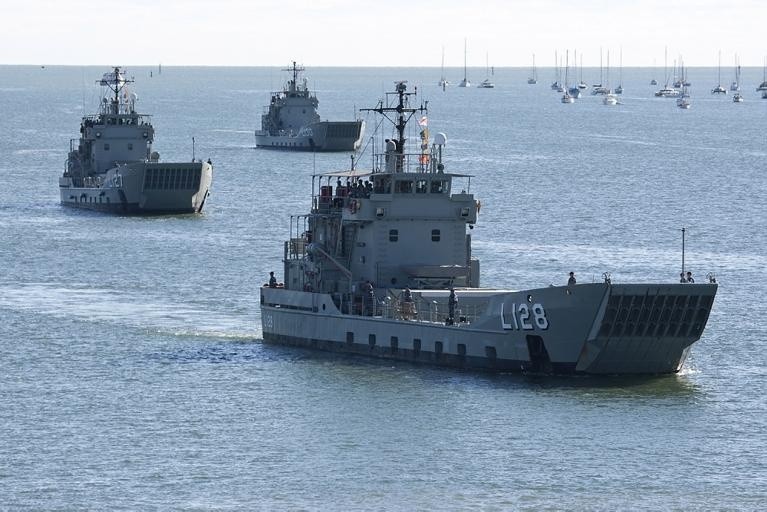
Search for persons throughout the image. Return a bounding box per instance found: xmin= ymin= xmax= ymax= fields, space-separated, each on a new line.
xmin=678 ymin=273 xmax=685 ymax=283
xmin=404 ymin=284 xmax=412 ymax=301
xmin=384 ymin=138 xmax=397 ymax=162
xmin=334 ymin=179 xmax=384 ymax=211
xmin=268 ymin=272 xmax=277 ymax=307
xmin=567 ymin=271 xmax=576 ymax=285
xmin=365 ymin=281 xmax=373 ymax=296
xmin=686 ymin=272 xmax=694 ymax=284
xmin=446 ymin=288 xmax=458 ymax=319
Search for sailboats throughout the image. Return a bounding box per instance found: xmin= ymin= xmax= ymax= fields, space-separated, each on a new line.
xmin=527 ymin=48 xmax=624 ymax=107
xmin=438 ymin=39 xmax=495 ymax=89
xmin=715 ymin=49 xmax=766 ymax=104
xmin=650 ymin=47 xmax=692 ymax=110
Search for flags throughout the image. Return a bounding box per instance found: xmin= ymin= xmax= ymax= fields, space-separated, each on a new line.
xmin=419 ymin=115 xmax=429 ymax=166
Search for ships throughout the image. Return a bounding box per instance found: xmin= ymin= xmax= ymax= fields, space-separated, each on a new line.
xmin=253 ymin=75 xmax=726 ymax=384
xmin=252 ymin=60 xmax=366 ymax=152
xmin=55 ymin=63 xmax=217 ymax=218
